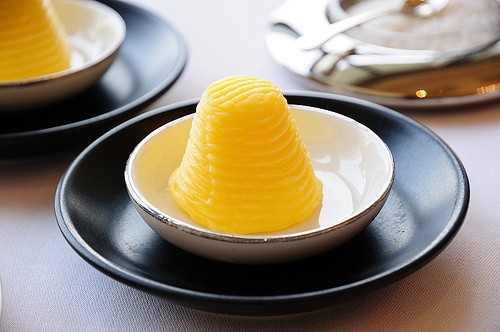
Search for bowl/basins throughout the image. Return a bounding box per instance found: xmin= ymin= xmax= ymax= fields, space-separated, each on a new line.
xmin=0 ymin=0 xmax=127 ymax=109
xmin=320 ymin=0 xmax=500 ymax=74
xmin=52 ymin=88 xmax=470 ymax=318
xmin=123 ymin=103 xmax=396 ymax=268
xmin=0 ymin=0 xmax=188 ymax=140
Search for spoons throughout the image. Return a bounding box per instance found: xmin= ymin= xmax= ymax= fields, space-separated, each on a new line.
xmin=292 ymin=0 xmax=453 ymax=53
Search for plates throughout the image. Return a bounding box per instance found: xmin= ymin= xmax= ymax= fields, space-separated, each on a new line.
xmin=256 ymin=0 xmax=500 ymax=110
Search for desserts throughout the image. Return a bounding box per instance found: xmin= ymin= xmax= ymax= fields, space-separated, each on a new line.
xmin=123 ymin=75 xmax=397 ymax=261
xmin=0 ymin=0 xmax=126 ymax=106
